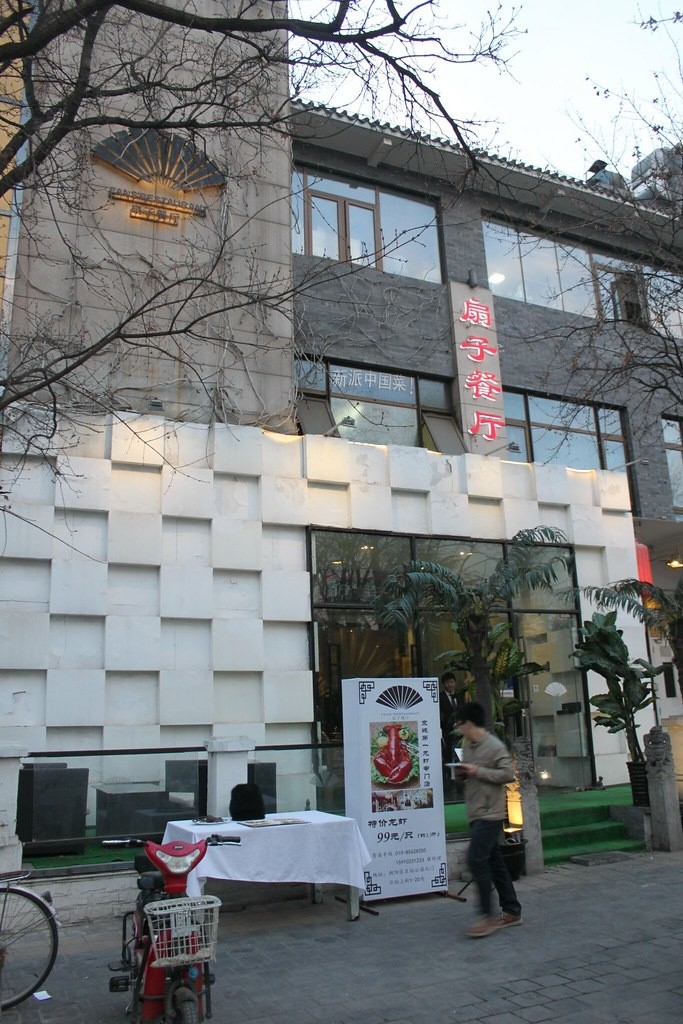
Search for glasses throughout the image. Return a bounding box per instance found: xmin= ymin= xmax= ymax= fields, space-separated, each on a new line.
xmin=453 ymin=722 xmax=464 ymax=729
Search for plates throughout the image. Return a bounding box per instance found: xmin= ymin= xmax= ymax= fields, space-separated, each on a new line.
xmin=190 ymin=818 xmax=229 ymax=825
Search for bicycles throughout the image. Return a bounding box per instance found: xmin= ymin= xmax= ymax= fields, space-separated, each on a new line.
xmin=0 ymin=867 xmax=64 ymax=1011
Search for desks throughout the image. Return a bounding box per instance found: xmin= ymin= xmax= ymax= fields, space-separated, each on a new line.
xmin=160 ymin=809 xmax=371 ymax=922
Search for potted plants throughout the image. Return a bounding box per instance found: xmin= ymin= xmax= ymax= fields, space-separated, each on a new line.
xmin=566 ymin=607 xmax=677 ymax=809
xmin=377 ymin=525 xmax=575 ymax=884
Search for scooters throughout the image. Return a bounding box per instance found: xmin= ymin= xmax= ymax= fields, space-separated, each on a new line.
xmin=102 ymin=830 xmax=242 ymax=1024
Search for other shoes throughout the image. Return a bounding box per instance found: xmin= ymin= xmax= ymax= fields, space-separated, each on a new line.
xmin=496 ymin=911 xmax=523 ymax=928
xmin=458 ymin=793 xmax=464 ymax=800
xmin=465 ymin=915 xmax=497 ymax=936
xmin=445 ymin=792 xmax=452 ymax=801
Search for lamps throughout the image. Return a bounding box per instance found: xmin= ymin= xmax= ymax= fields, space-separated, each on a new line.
xmin=466 ymin=269 xmax=478 ymax=287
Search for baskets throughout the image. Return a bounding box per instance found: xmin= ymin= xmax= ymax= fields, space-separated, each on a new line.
xmin=143 ymin=895 xmax=222 ymax=966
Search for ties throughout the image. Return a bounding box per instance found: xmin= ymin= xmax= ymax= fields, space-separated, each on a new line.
xmin=450 ymin=694 xmax=455 ymax=706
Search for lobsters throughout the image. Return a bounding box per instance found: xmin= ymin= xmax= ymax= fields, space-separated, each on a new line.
xmin=374 ymin=724 xmax=413 ymax=783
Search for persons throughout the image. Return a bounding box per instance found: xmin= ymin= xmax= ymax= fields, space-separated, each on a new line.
xmin=454 ymin=701 xmax=524 ymax=937
xmin=439 ymin=672 xmax=464 ymax=801
